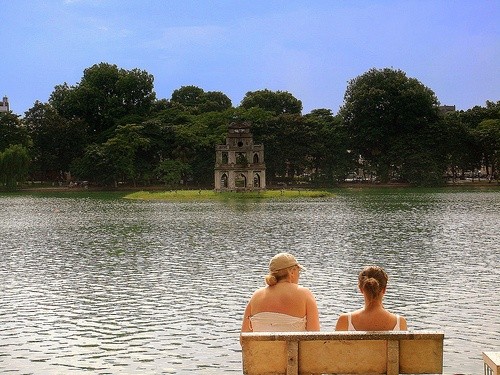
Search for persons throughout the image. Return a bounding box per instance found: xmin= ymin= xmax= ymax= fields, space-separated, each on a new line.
xmin=240 ymin=253 xmax=320 ymax=346
xmin=335 ymin=265 xmax=407 ymax=331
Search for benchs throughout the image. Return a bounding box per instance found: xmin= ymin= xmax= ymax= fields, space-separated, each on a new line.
xmin=240 ymin=330 xmax=444 ymax=375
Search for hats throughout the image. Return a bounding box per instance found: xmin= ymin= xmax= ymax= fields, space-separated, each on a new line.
xmin=269 ymin=253 xmax=307 ymax=273
